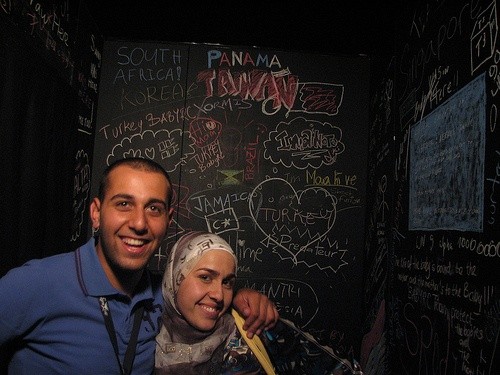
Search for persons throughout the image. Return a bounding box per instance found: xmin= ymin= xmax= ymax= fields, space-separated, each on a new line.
xmin=0 ymin=156 xmax=279 ymax=375
xmin=154 ymin=230 xmax=363 ymax=375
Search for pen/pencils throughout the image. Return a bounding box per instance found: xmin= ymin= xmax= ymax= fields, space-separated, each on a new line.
xmin=143 ymin=316 xmax=155 ymax=331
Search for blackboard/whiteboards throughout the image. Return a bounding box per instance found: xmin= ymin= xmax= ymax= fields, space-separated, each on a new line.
xmin=1 ymin=0 xmax=500 ymax=375
